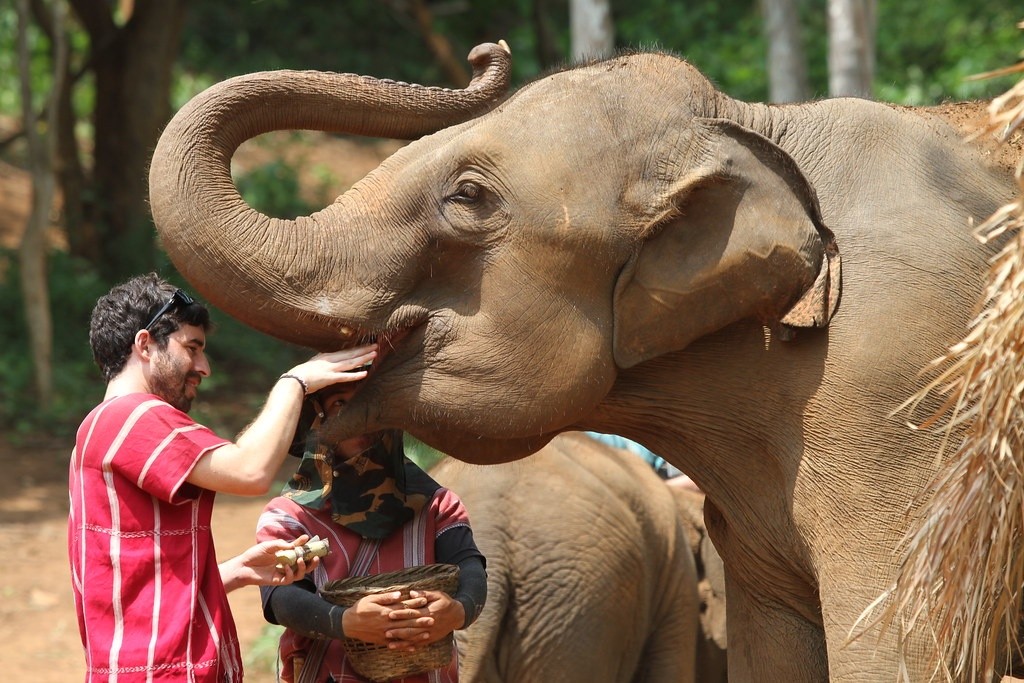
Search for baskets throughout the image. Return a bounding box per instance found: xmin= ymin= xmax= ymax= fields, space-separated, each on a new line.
xmin=318 ymin=563 xmax=460 ymax=683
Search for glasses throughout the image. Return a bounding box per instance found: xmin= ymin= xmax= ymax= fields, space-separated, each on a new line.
xmin=134 ymin=288 xmax=194 ymax=344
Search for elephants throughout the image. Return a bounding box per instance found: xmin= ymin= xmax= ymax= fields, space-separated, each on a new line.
xmin=149 ymin=37 xmax=1021 ymax=682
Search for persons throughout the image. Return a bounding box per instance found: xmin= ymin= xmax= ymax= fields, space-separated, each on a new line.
xmin=70 ymin=272 xmax=377 ymax=683
xmin=257 ymin=365 xmax=488 ymax=683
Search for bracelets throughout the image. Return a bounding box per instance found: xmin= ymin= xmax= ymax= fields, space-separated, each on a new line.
xmin=280 ymin=373 xmax=308 ymax=398
